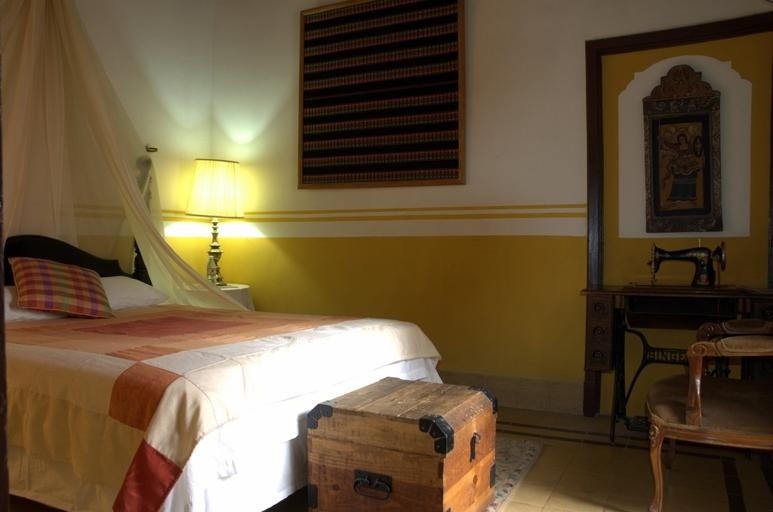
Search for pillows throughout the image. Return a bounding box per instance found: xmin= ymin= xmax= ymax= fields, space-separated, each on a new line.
xmin=8 ymin=256 xmax=118 ymax=319
xmin=4 ymin=285 xmax=69 ymax=322
xmin=99 ymin=275 xmax=171 ymax=312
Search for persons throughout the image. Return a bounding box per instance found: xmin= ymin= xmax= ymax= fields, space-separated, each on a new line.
xmin=661 ymin=123 xmax=705 ymax=203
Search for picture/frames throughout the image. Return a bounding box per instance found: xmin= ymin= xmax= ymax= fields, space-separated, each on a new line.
xmin=642 ymin=64 xmax=723 ymax=233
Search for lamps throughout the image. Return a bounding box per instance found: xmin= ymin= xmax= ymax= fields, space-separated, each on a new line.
xmin=183 ymin=158 xmax=246 ymax=286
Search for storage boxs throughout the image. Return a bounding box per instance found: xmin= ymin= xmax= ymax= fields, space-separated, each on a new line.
xmin=306 ymin=376 xmax=498 ymax=512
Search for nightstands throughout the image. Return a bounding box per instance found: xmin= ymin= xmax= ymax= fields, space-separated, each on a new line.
xmin=202 ymin=284 xmax=256 ymax=313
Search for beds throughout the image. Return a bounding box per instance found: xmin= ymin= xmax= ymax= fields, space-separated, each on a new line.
xmin=0 ymin=234 xmax=443 ymax=512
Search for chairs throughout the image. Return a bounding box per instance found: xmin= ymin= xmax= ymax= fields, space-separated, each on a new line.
xmin=644 ymin=316 xmax=773 ymax=512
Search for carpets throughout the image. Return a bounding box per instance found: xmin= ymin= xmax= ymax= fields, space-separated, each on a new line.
xmin=482 ymin=428 xmax=544 ymax=512
